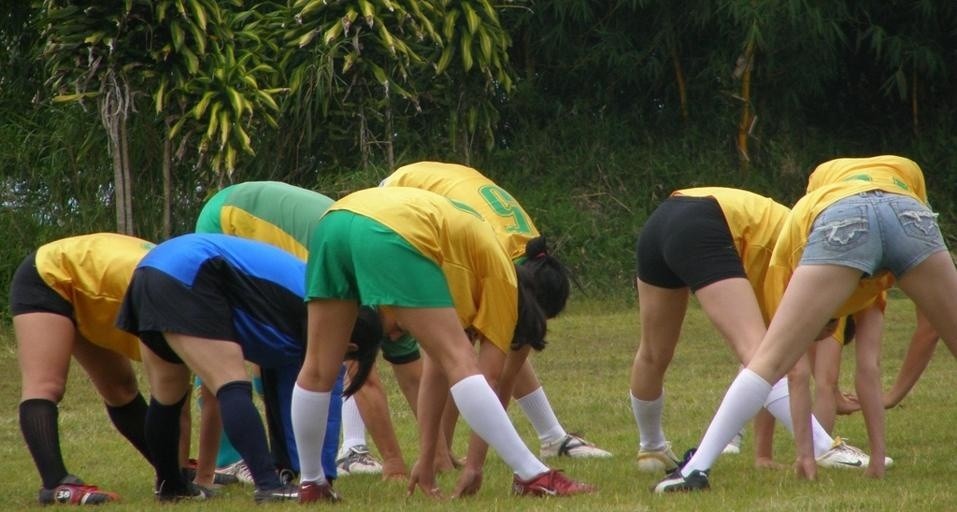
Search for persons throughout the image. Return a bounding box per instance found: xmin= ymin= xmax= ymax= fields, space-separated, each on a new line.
xmin=286 ymin=180 xmax=601 ymax=502
xmin=7 ymin=232 xmax=238 ymax=504
xmin=625 ymin=189 xmax=894 ymax=474
xmin=805 ymin=154 xmax=940 ymax=416
xmin=646 ymin=181 xmax=956 ymax=496
xmin=111 ymin=233 xmax=382 ymax=501
xmin=375 ymin=158 xmax=614 ymax=472
xmin=192 ymin=176 xmax=457 ymax=485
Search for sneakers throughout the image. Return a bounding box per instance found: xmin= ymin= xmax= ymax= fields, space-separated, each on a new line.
xmin=637 ymin=441 xmax=681 ymax=472
xmin=215 ymin=460 xmax=252 ymax=481
xmin=180 ymin=465 xmax=238 ymax=484
xmin=511 ymin=469 xmax=596 ymax=496
xmin=540 ymin=433 xmax=610 ymax=457
xmin=38 ymin=475 xmax=119 ymax=504
xmin=652 ymin=448 xmax=711 ymax=492
xmin=298 ymin=478 xmax=340 ymax=502
xmin=815 ymin=436 xmax=894 ymax=467
xmin=155 ymin=480 xmax=211 ymax=500
xmin=255 ymin=484 xmax=300 ymax=502
xmin=336 ymin=445 xmax=382 ymax=473
